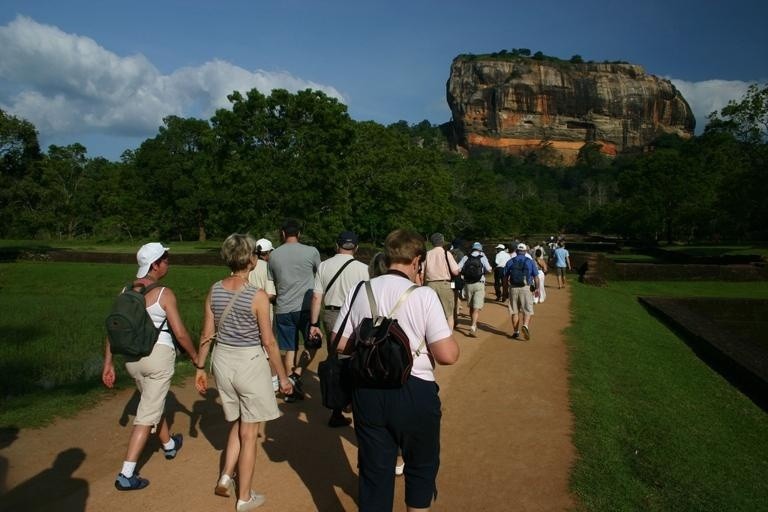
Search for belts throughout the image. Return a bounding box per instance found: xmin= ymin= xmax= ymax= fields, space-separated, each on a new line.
xmin=428 ymin=280 xmax=451 ymax=281
xmin=325 ymin=306 xmax=340 ymax=310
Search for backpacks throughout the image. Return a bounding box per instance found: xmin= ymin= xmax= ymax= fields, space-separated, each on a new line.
xmin=337 ymin=282 xmax=426 ymax=389
xmin=106 ymin=281 xmax=167 ymax=357
xmin=510 ymin=258 xmax=529 ymax=286
xmin=462 ymin=254 xmax=484 ymax=282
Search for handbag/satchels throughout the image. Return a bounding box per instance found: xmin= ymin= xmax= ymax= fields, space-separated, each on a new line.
xmin=318 ymin=355 xmax=351 ymax=409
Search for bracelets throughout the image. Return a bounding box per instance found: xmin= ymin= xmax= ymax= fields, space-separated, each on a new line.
xmin=309 ymin=322 xmax=321 ymax=327
xmin=196 ymin=364 xmax=206 ymax=370
xmin=279 ymin=379 xmax=290 ymax=385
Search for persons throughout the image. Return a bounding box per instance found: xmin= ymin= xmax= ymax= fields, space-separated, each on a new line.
xmin=228 ymin=237 xmax=278 ymax=362
xmin=327 ymin=227 xmax=462 ymax=512
xmin=394 ymin=448 xmax=408 ymax=476
xmin=266 ymin=221 xmax=322 ymax=402
xmin=193 ymin=231 xmax=296 ymax=511
xmin=101 ymin=241 xmax=201 ymax=491
xmin=367 ymin=231 xmax=572 ymax=339
xmin=308 ymin=229 xmax=370 ymax=427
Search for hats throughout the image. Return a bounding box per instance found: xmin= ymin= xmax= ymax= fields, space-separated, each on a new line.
xmin=472 ymin=242 xmax=482 ymax=250
xmin=337 ymin=232 xmax=357 ymax=250
xmin=517 ymin=243 xmax=527 ymax=250
xmin=136 ymin=242 xmax=169 ymax=278
xmin=256 ymin=238 xmax=274 ymax=251
xmin=494 ymin=244 xmax=505 ymax=249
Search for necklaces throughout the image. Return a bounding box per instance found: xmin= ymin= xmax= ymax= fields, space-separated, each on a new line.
xmin=230 ymin=272 xmax=250 ymax=282
xmin=142 ymin=274 xmax=157 ymax=282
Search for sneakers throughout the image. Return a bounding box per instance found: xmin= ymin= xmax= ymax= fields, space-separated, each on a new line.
xmin=164 ymin=434 xmax=182 ymax=459
xmin=329 ymin=414 xmax=352 ymax=427
xmin=215 ymin=474 xmax=232 ymax=497
xmin=272 ymin=374 xmax=304 ymax=402
xmin=470 ymin=324 xmax=478 ymax=336
xmin=115 ymin=473 xmax=149 ymax=490
xmin=237 ymin=494 xmax=264 ymax=511
xmin=513 ymin=326 xmax=529 ymax=340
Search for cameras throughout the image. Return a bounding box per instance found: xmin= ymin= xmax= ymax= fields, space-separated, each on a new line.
xmin=300 ymin=333 xmax=322 ymax=367
